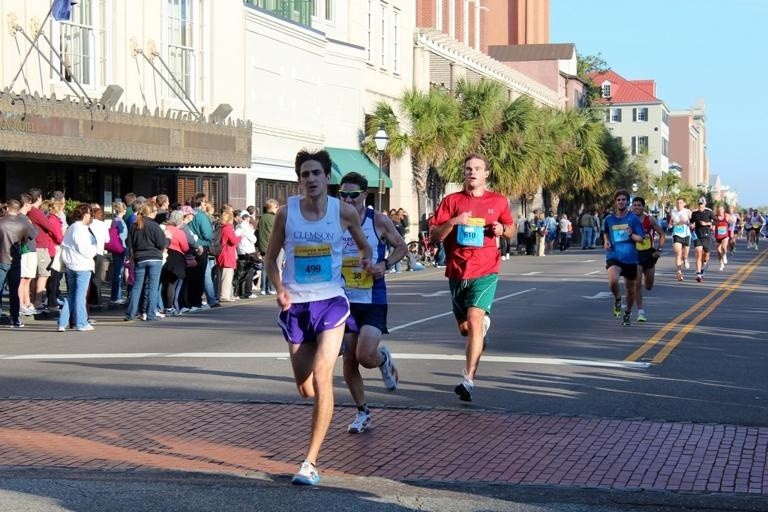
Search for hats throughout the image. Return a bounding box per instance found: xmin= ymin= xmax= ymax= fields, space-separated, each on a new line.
xmin=697 ymin=196 xmax=707 ymax=205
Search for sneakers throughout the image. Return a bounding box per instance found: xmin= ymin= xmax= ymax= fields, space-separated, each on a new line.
xmin=453 ymin=376 xmax=475 ymax=403
xmin=613 ymin=302 xmax=622 ymax=319
xmin=376 ymin=347 xmax=401 ymax=394
xmin=622 ymin=310 xmax=632 ymax=326
xmin=0 ymin=282 xmax=277 ymax=332
xmin=500 ymin=240 xmax=599 ymax=262
xmin=480 ymin=315 xmax=491 ymax=338
xmin=673 ymin=234 xmax=759 ymax=282
xmin=388 ymin=259 xmax=447 ymax=274
xmin=637 ymin=310 xmax=648 ymax=322
xmin=347 ymin=409 xmax=372 ymax=435
xmin=288 ymin=459 xmax=320 ymax=486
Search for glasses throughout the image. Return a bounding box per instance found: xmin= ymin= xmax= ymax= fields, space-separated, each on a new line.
xmin=337 ymin=188 xmax=365 ymax=199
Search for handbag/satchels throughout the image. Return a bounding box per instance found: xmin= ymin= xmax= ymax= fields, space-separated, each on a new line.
xmin=182 ymin=253 xmax=198 ymax=267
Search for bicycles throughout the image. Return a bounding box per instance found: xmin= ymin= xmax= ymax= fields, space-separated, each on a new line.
xmin=406 ymin=230 xmax=437 ymax=261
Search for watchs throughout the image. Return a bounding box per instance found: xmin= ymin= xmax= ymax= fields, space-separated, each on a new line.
xmin=382 ymin=258 xmax=391 ymax=271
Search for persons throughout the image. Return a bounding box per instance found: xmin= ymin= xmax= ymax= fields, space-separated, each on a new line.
xmin=262 ymin=144 xmax=374 ymax=485
xmin=1 ymin=187 xmax=283 ymax=333
xmin=334 ymin=172 xmax=408 ymax=434
xmin=428 ymin=152 xmax=517 ymax=403
xmin=381 ymin=204 xmax=601 ymax=277
xmin=601 ymin=187 xmax=768 ymax=326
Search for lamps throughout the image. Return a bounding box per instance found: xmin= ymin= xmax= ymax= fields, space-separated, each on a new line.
xmin=208 ymin=103 xmax=233 ymax=125
xmin=99 ymin=84 xmax=124 ymax=111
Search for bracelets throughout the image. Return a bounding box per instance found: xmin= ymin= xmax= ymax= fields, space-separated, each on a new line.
xmin=360 ymin=256 xmax=367 ymax=260
xmin=501 ymin=225 xmax=506 ymax=234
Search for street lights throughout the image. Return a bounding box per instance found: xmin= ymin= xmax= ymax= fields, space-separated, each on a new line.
xmin=653 ymin=186 xmax=659 ymax=213
xmin=632 ymin=182 xmax=638 ymax=198
xmin=372 ymin=127 xmax=392 ymax=213
